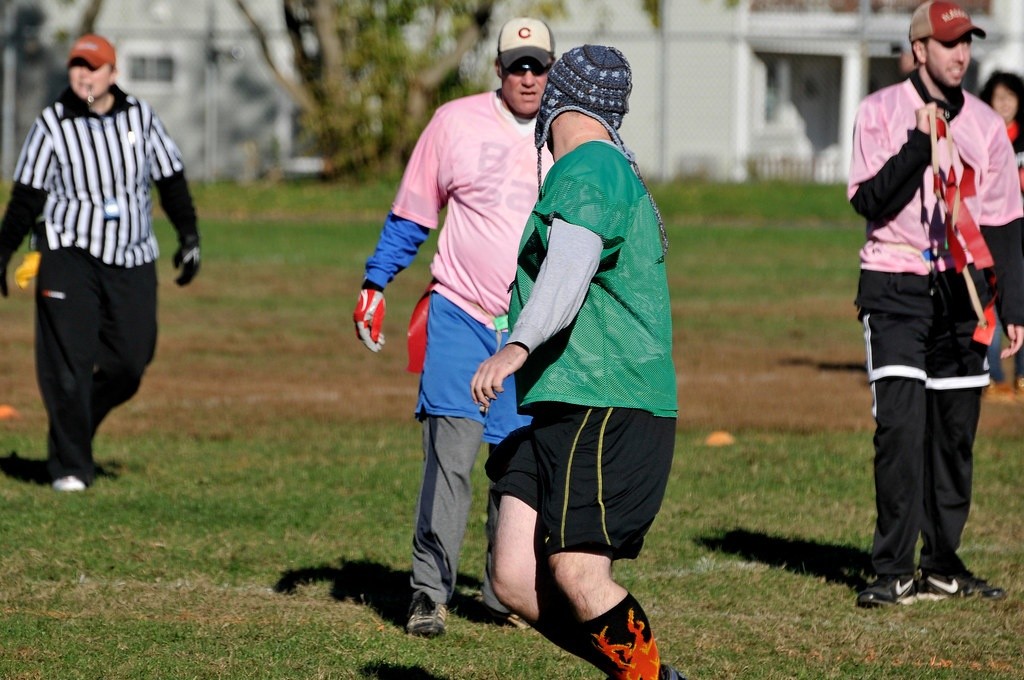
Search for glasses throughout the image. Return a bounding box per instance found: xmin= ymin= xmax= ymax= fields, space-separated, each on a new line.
xmin=506 ymin=57 xmax=551 ymax=76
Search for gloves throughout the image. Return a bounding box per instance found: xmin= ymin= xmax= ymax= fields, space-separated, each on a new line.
xmin=351 ymin=276 xmax=386 ymax=353
xmin=173 ymin=236 xmax=201 ymax=286
xmin=0 ymin=248 xmax=8 ymax=298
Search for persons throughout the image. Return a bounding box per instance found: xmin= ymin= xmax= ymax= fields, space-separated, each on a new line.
xmin=351 ymin=18 xmax=554 ymax=642
xmin=0 ymin=32 xmax=203 ymax=493
xmin=846 ymin=0 xmax=1024 ymax=609
xmin=470 ymin=44 xmax=691 ymax=680
xmin=969 ymin=70 xmax=1024 ymax=401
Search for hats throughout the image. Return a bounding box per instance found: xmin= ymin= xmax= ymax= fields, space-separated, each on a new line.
xmin=535 ymin=45 xmax=671 ymax=263
xmin=909 ymin=0 xmax=986 ymax=43
xmin=497 ymin=16 xmax=555 ymax=69
xmin=67 ymin=33 xmax=116 ymax=69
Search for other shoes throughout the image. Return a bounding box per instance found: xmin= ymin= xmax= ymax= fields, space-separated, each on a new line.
xmin=658 ymin=664 xmax=687 ymax=680
xmin=51 ymin=476 xmax=87 ymax=492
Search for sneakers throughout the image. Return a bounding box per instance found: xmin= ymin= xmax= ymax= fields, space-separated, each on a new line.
xmin=483 ymin=597 xmax=533 ymax=629
xmin=856 ymin=575 xmax=918 ymax=606
xmin=405 ymin=596 xmax=448 ymax=639
xmin=916 ymin=564 xmax=1006 ymax=605
